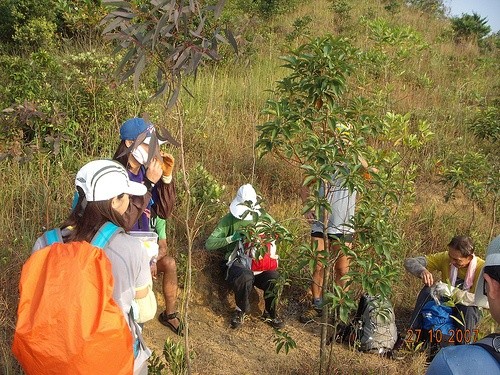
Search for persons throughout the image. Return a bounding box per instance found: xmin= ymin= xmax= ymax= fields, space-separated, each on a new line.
xmin=113 ymin=117 xmax=193 ymax=338
xmin=27 ymin=159 xmax=159 ymax=375
xmin=424 ymin=236 xmax=500 ymax=375
xmin=385 ymin=234 xmax=488 ymax=365
xmin=297 ymin=119 xmax=379 ymax=323
xmin=203 ymin=183 xmax=287 ymax=329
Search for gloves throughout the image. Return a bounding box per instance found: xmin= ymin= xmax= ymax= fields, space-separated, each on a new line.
xmin=226 ymin=231 xmax=242 ymax=244
xmin=159 ymin=151 xmax=175 ymax=175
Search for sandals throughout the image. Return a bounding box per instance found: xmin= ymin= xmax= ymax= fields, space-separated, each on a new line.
xmin=158 ymin=310 xmax=190 ymax=336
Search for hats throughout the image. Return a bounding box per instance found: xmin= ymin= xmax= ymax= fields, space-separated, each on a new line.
xmin=334 ymin=123 xmax=355 ymax=148
xmin=229 ymin=183 xmax=262 ymax=220
xmin=119 ymin=117 xmax=166 ymax=146
xmin=73 ymin=160 xmax=147 ymax=201
xmin=473 ymin=235 xmax=500 ymax=305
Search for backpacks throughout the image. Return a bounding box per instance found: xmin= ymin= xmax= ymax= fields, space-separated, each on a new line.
xmin=227 ymin=222 xmax=279 ymax=271
xmin=11 ymin=222 xmax=133 ymax=375
xmin=352 ymin=295 xmax=397 ymax=355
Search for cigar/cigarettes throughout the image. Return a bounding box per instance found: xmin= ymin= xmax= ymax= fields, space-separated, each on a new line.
xmin=153 ymin=276 xmax=158 ymax=281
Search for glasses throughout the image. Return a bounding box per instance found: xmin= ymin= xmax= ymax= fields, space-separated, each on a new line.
xmin=483 ymin=279 xmax=487 ymax=297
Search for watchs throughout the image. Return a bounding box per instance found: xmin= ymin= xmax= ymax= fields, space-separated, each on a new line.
xmin=151 ymin=258 xmax=159 ymax=264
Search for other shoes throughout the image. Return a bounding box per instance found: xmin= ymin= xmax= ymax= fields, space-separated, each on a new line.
xmin=393 ymin=344 xmax=417 ymax=359
xmin=425 ymin=348 xmax=438 ymax=367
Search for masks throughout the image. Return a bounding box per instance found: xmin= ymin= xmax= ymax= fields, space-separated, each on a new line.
xmin=127 ymin=140 xmax=149 ymax=165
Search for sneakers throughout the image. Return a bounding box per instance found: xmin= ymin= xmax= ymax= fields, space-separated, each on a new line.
xmin=261 ymin=309 xmax=284 ymax=329
xmin=299 ymin=304 xmax=322 ymax=324
xmin=229 ymin=305 xmax=247 ymax=328
